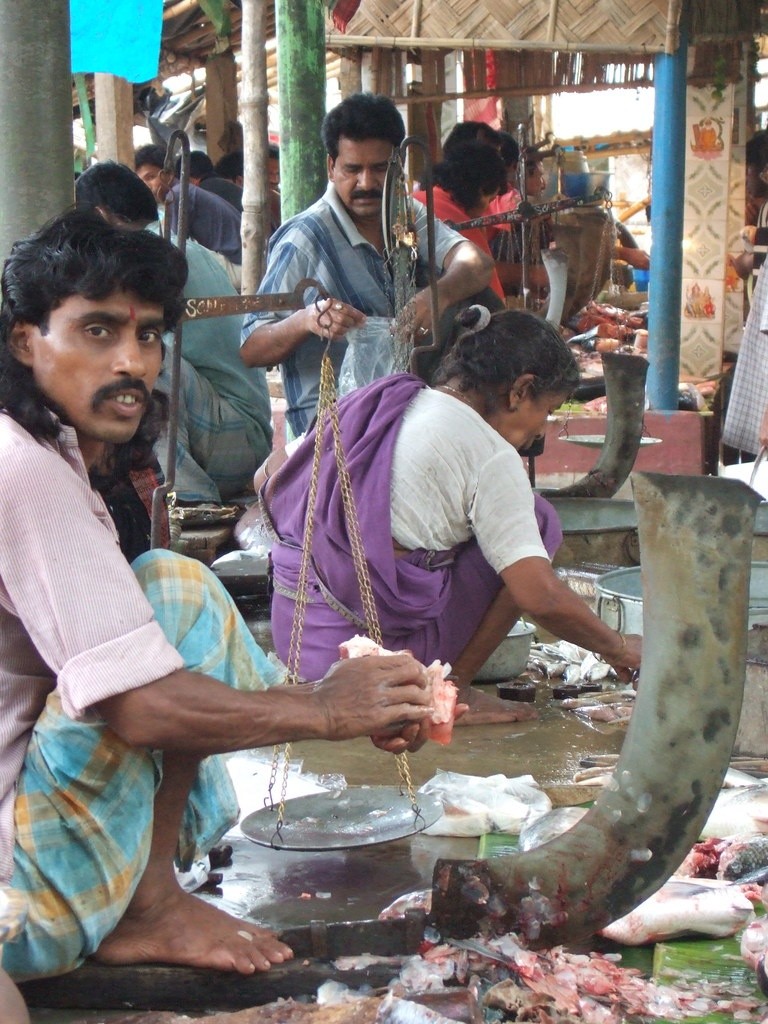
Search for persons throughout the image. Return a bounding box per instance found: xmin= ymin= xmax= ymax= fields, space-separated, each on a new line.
xmin=75 ymin=91 xmax=651 ymax=505
xmin=0 ymin=962 xmax=32 ymax=1024
xmin=720 ymin=129 xmax=768 ymax=455
xmin=257 ymin=302 xmax=644 ymax=727
xmin=0 ymin=201 xmax=471 ymax=975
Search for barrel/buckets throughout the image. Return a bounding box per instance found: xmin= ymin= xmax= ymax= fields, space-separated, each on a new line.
xmin=593 ymin=562 xmax=768 ymax=639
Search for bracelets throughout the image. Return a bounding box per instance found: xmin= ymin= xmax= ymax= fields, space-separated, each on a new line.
xmin=600 ymin=630 xmax=627 ymax=664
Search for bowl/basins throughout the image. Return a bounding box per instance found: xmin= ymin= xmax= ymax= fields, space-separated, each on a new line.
xmin=471 ymin=621 xmax=537 ymax=682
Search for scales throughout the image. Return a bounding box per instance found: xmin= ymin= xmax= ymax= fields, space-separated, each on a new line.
xmin=150 ymin=129 xmax=445 ymax=852
xmin=443 ymin=123 xmax=664 ymax=447
xmin=382 ymin=134 xmax=441 ymax=374
